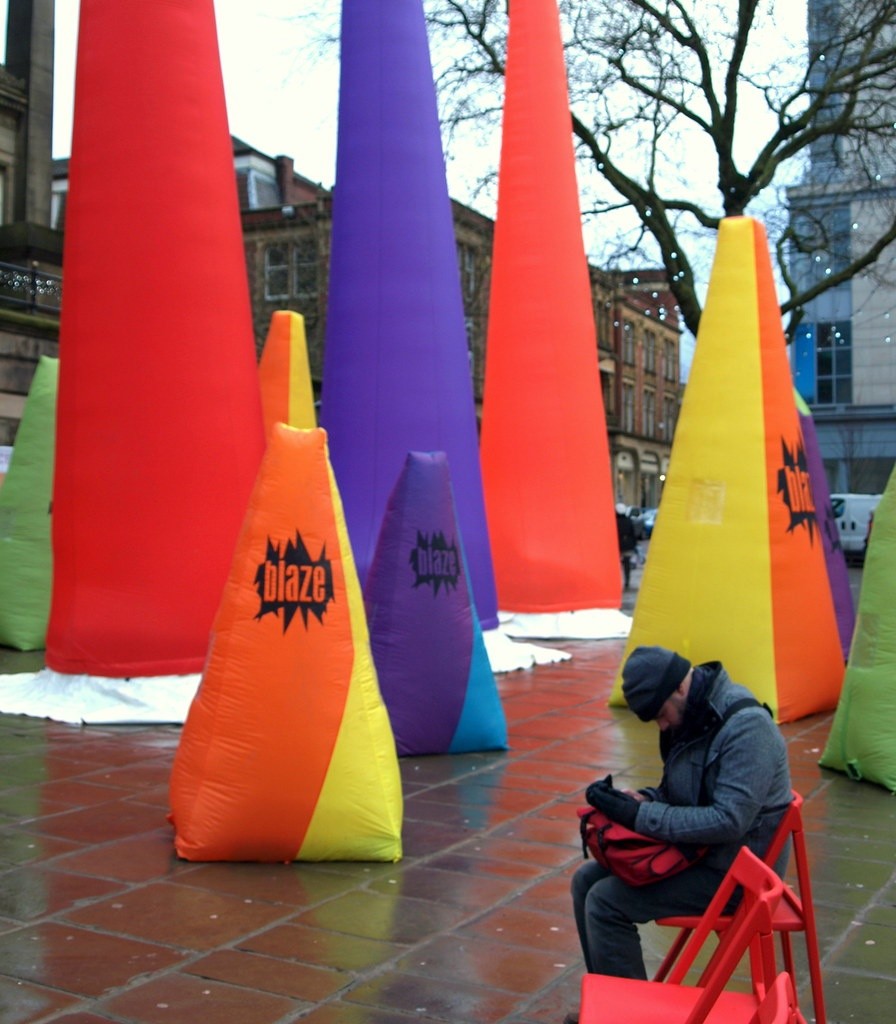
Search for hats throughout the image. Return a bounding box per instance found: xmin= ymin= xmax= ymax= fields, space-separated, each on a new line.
xmin=622 ymin=646 xmax=692 ymax=722
xmin=616 ymin=503 xmax=627 ymax=514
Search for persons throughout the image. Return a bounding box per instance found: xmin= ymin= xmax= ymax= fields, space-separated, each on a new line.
xmin=572 ymin=646 xmax=793 ymax=1024
xmin=615 ymin=512 xmax=639 ymax=589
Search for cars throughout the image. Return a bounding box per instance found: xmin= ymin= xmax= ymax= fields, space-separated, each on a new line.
xmin=634 ymin=507 xmax=659 ymax=540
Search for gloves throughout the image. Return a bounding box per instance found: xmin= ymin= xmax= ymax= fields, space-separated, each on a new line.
xmin=586 ymin=774 xmax=640 ymax=831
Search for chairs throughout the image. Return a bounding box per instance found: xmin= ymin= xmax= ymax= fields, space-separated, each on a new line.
xmin=576 ymin=844 xmax=808 ymax=1024
xmin=651 ymin=789 xmax=827 ymax=1024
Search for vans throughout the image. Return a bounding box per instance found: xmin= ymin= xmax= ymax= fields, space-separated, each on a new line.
xmin=831 ymin=493 xmax=880 ymax=560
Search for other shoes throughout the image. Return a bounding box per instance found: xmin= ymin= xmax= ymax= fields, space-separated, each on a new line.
xmin=563 ymin=1012 xmax=579 ymax=1024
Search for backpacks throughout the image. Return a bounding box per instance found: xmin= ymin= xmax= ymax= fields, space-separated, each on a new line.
xmin=576 ymin=803 xmax=708 ymax=887
